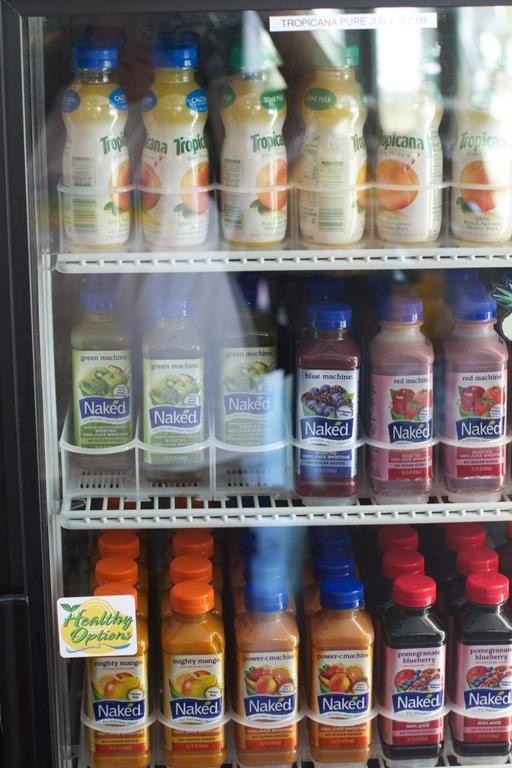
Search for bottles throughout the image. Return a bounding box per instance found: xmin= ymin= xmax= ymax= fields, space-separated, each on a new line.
xmin=59 ymin=32 xmax=511 ymax=249
xmin=61 ymin=270 xmax=505 ymax=504
xmin=78 ymin=522 xmax=510 ymax=767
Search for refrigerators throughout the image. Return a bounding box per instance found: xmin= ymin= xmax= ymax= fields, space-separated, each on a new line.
xmin=2 ymin=0 xmax=510 ymax=766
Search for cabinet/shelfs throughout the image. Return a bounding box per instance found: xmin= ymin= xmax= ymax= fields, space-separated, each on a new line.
xmin=49 ymin=218 xmax=511 ymax=763
